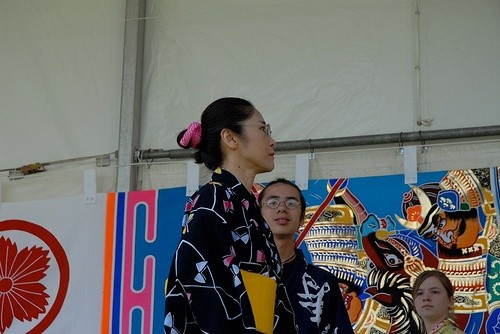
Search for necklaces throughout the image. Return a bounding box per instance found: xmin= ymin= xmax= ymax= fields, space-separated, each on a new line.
xmin=281 ymin=254 xmax=295 ymax=263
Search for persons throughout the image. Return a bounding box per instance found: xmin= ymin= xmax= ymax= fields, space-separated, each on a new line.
xmin=412 ymin=270 xmax=465 ymax=334
xmin=257 ymin=178 xmax=354 ymax=334
xmin=164 ymin=97 xmax=298 ymax=334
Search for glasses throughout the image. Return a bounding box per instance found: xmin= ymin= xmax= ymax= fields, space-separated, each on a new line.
xmin=261 ymin=199 xmax=302 ymax=210
xmin=231 ymin=124 xmax=272 ymax=136
